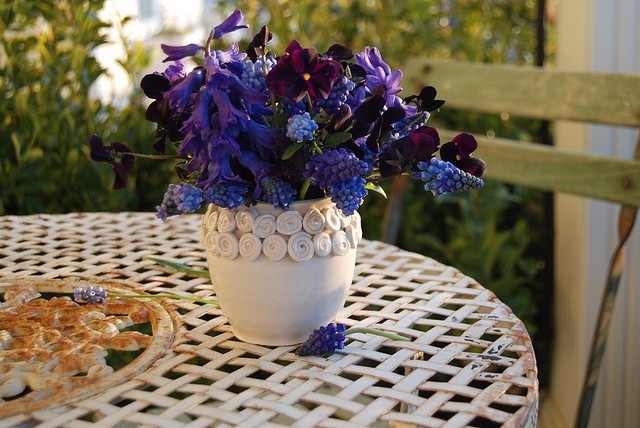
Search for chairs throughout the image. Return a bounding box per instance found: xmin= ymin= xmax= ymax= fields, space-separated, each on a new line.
xmin=379 ymin=54 xmax=639 ymax=419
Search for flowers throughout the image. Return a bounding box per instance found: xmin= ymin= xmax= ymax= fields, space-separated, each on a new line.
xmin=140 ymin=8 xmax=486 ymax=217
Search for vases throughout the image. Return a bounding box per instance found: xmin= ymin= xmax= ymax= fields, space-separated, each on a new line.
xmin=200 ymin=198 xmax=362 ymax=346
